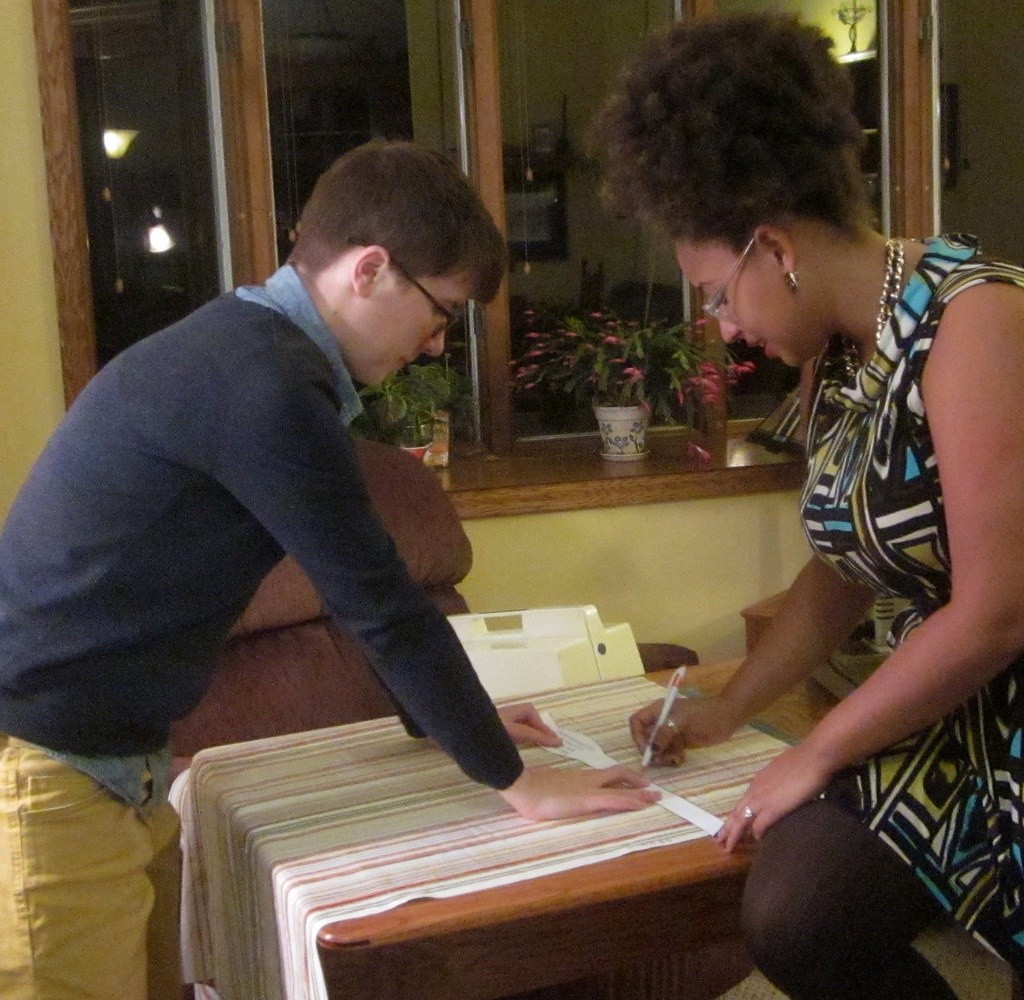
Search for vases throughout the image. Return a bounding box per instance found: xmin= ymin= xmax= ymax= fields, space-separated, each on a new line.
xmin=593 ymin=403 xmax=651 ymax=460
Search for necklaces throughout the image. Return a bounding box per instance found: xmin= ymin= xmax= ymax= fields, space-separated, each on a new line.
xmin=842 ymin=240 xmax=905 ymax=379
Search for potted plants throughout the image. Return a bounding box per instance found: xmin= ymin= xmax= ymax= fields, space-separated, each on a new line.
xmin=357 ymin=362 xmax=460 ymax=468
xmin=507 ymin=309 xmax=756 ymax=462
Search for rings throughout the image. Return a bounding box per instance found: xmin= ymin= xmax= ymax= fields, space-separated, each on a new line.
xmin=741 ymin=805 xmax=753 ymax=820
xmin=666 ymin=718 xmax=679 ymax=735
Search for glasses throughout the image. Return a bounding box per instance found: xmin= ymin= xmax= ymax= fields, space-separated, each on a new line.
xmin=344 ymin=235 xmax=463 ymax=340
xmin=702 ymin=238 xmax=755 ymax=327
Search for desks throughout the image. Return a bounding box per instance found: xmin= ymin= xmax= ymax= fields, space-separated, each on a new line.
xmin=193 ymin=659 xmax=841 ymax=1000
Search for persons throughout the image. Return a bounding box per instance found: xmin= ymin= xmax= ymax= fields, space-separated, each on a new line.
xmin=584 ymin=13 xmax=1024 ymax=999
xmin=2 ymin=140 xmax=665 ymax=999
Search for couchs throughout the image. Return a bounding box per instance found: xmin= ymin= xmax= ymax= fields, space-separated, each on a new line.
xmin=169 ymin=438 xmax=475 ymax=790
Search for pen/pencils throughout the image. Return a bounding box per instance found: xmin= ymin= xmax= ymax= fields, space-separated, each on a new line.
xmin=640 ymin=664 xmax=687 ymax=775
